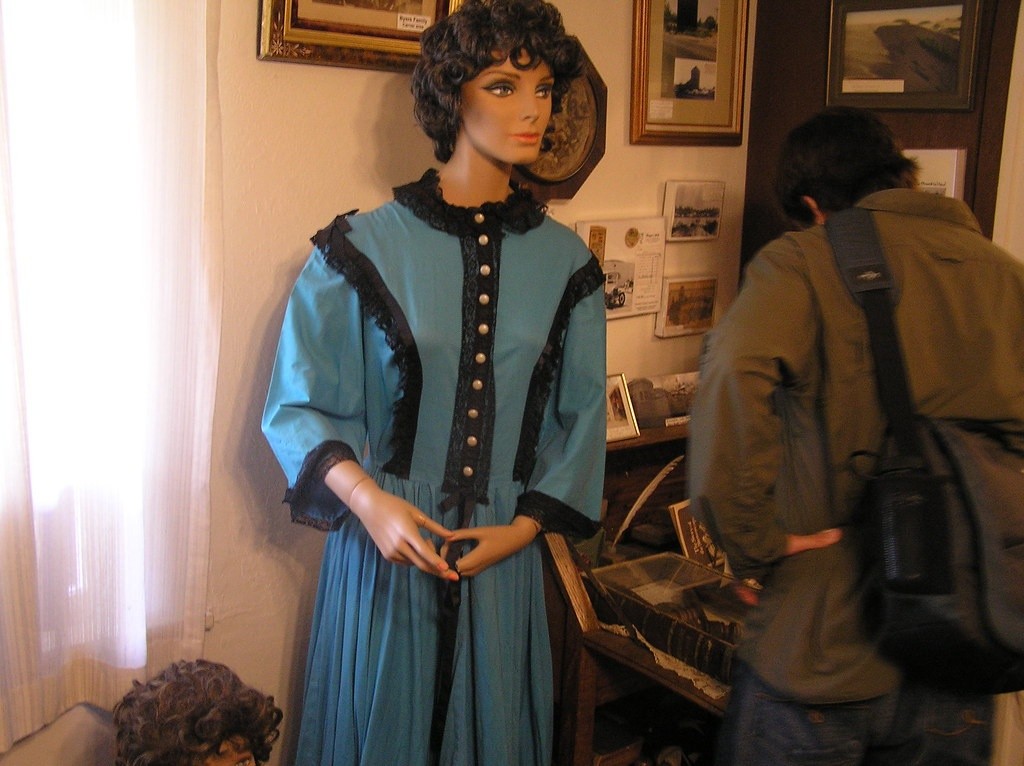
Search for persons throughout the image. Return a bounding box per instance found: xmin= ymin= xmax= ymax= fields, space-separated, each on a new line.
xmin=686 ymin=105 xmax=1024 ymax=766
xmin=111 ymin=0 xmax=617 ymax=766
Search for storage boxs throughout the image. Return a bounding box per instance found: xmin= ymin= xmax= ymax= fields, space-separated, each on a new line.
xmin=581 ymin=551 xmax=764 ymax=684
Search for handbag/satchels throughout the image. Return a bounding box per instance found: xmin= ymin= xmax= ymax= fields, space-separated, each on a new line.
xmin=856 ymin=411 xmax=1024 ymax=694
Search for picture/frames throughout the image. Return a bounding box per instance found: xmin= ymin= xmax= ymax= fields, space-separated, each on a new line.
xmin=606 ymin=373 xmax=641 ymax=443
xmin=825 ymin=0 xmax=984 ymax=112
xmin=654 ymin=276 xmax=720 ymax=338
xmin=509 ymin=35 xmax=607 ymax=199
xmin=629 ymin=0 xmax=751 ymax=148
xmin=254 ymin=0 xmax=466 ymax=76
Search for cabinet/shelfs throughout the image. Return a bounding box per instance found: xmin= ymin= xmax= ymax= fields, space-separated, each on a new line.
xmin=541 ymin=414 xmax=744 ymax=766
